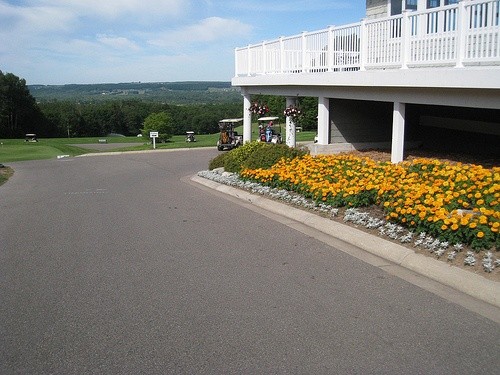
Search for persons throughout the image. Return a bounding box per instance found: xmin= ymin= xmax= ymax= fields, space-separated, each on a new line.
xmin=266 ymin=126 xmax=272 ymax=136
xmin=221 ymin=128 xmax=236 ymax=144
